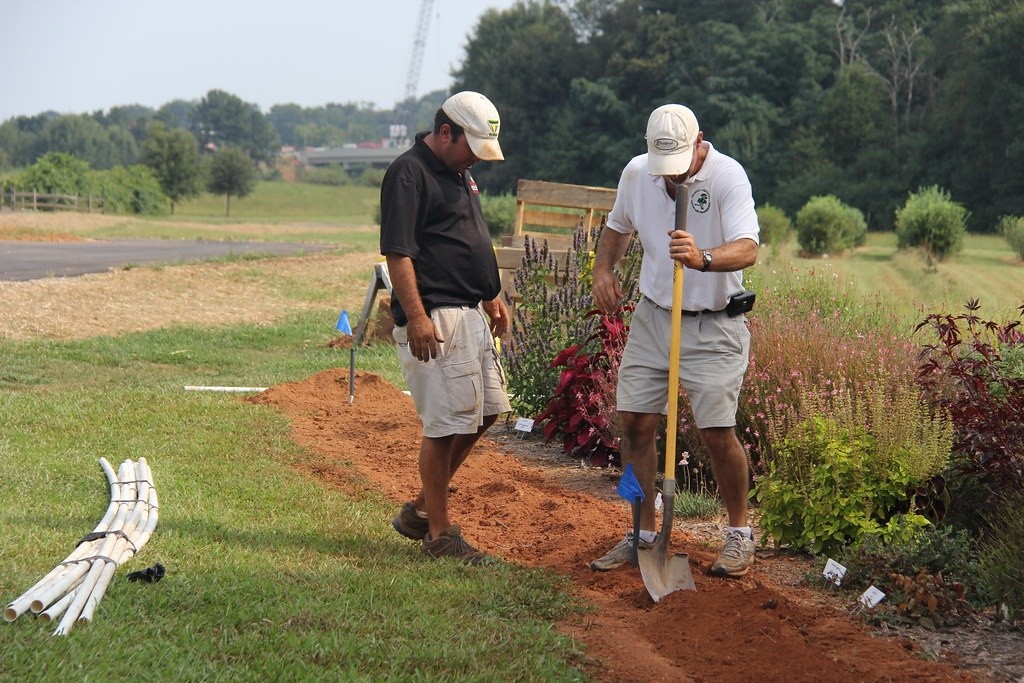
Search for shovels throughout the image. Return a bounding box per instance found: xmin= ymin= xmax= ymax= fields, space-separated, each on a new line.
xmin=636 ymin=185 xmax=699 ymax=604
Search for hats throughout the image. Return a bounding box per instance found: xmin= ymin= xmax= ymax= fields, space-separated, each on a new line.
xmin=442 ymin=91 xmax=505 ymax=160
xmin=646 ymin=104 xmax=699 ymax=175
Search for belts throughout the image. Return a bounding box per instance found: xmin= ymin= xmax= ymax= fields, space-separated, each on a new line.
xmin=645 ymin=296 xmax=724 ymax=316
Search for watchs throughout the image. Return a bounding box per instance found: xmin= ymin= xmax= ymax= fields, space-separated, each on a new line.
xmin=699 ymin=249 xmax=713 ymax=273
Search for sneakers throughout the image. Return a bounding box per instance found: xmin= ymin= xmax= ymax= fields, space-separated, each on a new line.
xmin=392 ymin=500 xmax=429 ymax=541
xmin=591 ymin=531 xmax=661 ymax=572
xmin=422 ymin=525 xmax=493 ymax=567
xmin=712 ymin=530 xmax=757 ymax=575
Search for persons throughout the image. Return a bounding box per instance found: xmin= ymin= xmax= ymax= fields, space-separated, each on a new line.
xmin=590 ymin=103 xmax=763 ymax=579
xmin=379 ymin=90 xmax=521 ymax=569
